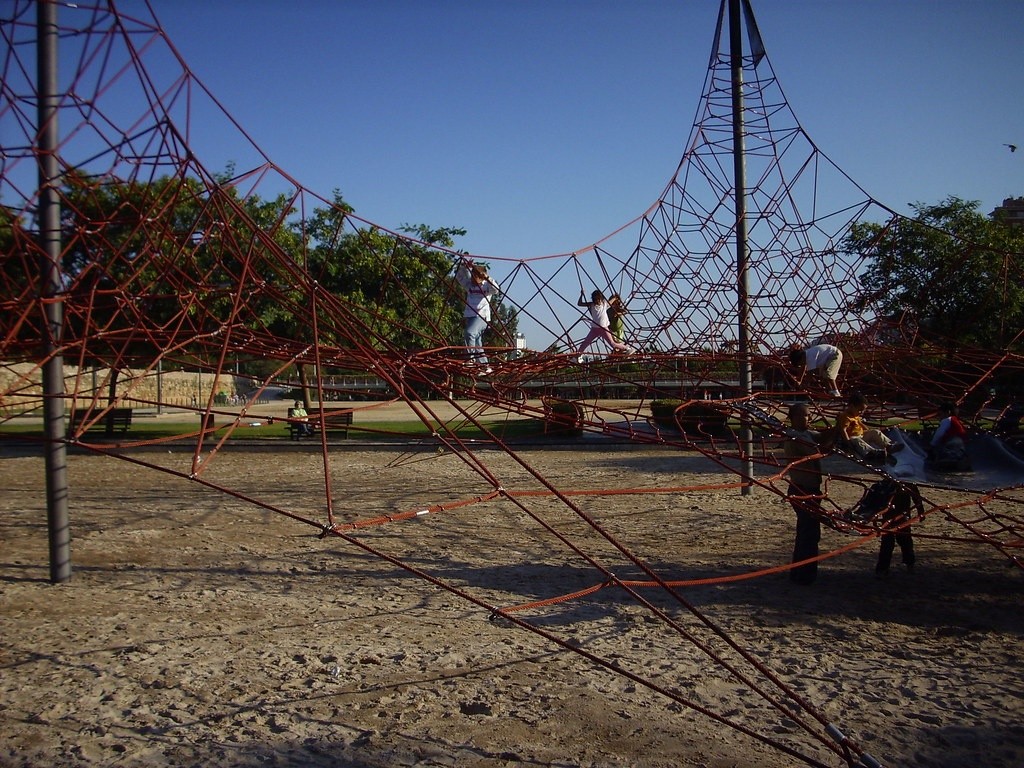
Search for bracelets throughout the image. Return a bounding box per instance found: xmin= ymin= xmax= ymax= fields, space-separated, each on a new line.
xmin=487 ymin=276 xmax=489 ymax=279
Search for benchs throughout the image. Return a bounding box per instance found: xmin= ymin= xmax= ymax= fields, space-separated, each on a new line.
xmin=70 ymin=408 xmax=134 ymax=439
xmin=918 ymin=406 xmax=941 ymax=429
xmin=284 ymin=407 xmax=353 ymax=439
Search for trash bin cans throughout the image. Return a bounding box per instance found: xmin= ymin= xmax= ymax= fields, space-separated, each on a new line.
xmin=201 ymin=414 xmax=214 ymax=440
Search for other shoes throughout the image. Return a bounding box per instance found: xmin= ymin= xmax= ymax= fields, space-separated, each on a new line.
xmin=887 ymin=443 xmax=906 ymax=453
xmin=571 ymin=356 xmax=583 ymax=363
xmin=882 ymin=448 xmax=888 ymax=464
xmin=463 ymin=357 xmax=475 ymax=365
xmin=303 ymin=430 xmax=311 ymax=435
xmin=830 ymin=391 xmax=840 ymax=397
xmin=626 ymin=348 xmax=635 ymax=357
xmin=477 ymin=367 xmax=493 ymax=377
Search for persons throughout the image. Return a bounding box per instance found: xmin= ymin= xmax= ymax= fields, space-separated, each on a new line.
xmin=577 ymin=289 xmax=634 ymax=355
xmin=840 ymin=393 xmax=906 ymax=457
xmin=783 ymin=403 xmax=840 ymax=584
xmin=790 ymin=344 xmax=843 ymax=396
xmin=456 ymin=258 xmax=499 ymax=374
xmin=924 ymin=403 xmax=967 ymax=470
xmin=291 ymin=401 xmax=311 ymax=441
xmin=873 ymin=478 xmax=926 ymax=579
